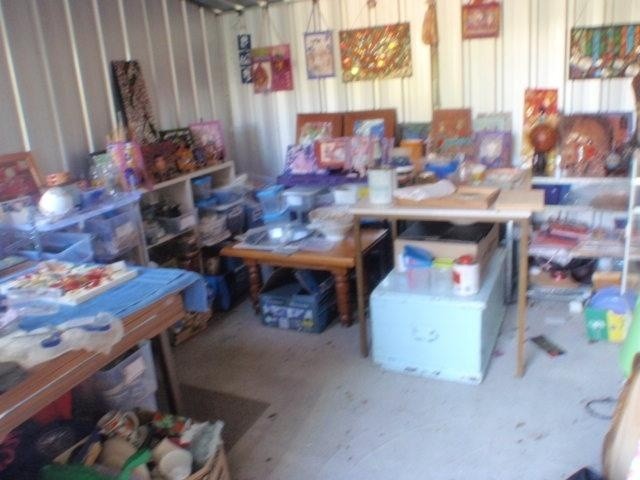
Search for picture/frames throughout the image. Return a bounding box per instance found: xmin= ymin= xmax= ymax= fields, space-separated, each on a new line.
xmin=296 ymin=109 xmax=395 ymax=174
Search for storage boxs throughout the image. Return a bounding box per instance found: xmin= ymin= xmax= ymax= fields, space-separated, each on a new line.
xmin=12 ymin=208 xmax=139 ymax=263
xmin=81 ymin=339 xmax=160 ymax=415
xmin=391 ymin=223 xmax=500 ymax=274
xmin=369 ymin=250 xmax=507 ymax=384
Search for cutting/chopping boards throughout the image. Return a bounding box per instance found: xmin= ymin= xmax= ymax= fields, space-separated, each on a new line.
xmin=396 ymin=185 xmax=501 ymax=209
xmin=9 ymin=260 xmax=139 ymax=305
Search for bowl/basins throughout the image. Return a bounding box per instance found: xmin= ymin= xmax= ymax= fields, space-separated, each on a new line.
xmin=308 ymin=206 xmax=356 ymax=241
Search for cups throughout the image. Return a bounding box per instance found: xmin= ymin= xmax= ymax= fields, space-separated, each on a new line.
xmin=97 ymin=406 xmax=140 ymax=445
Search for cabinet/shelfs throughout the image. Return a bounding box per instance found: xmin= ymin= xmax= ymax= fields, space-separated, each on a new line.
xmin=0 ymin=161 xmax=237 ymax=344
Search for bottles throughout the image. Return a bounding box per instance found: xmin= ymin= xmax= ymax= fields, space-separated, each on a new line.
xmin=86 ymin=150 xmax=118 ymax=199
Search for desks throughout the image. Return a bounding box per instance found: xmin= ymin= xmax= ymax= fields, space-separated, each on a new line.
xmin=222 ymin=223 xmax=385 ymax=330
xmin=1 ymin=268 xmax=185 ymax=442
xmin=348 ymin=187 xmax=547 ymax=378
